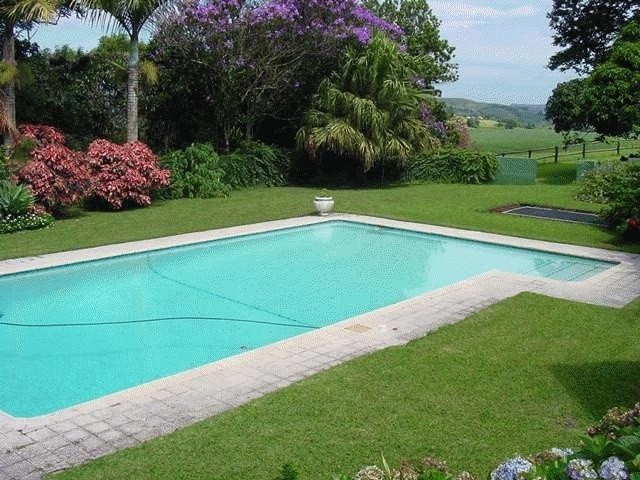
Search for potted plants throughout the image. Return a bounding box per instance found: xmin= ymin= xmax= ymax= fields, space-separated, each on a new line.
xmin=313 ymin=188 xmax=334 ymax=216
